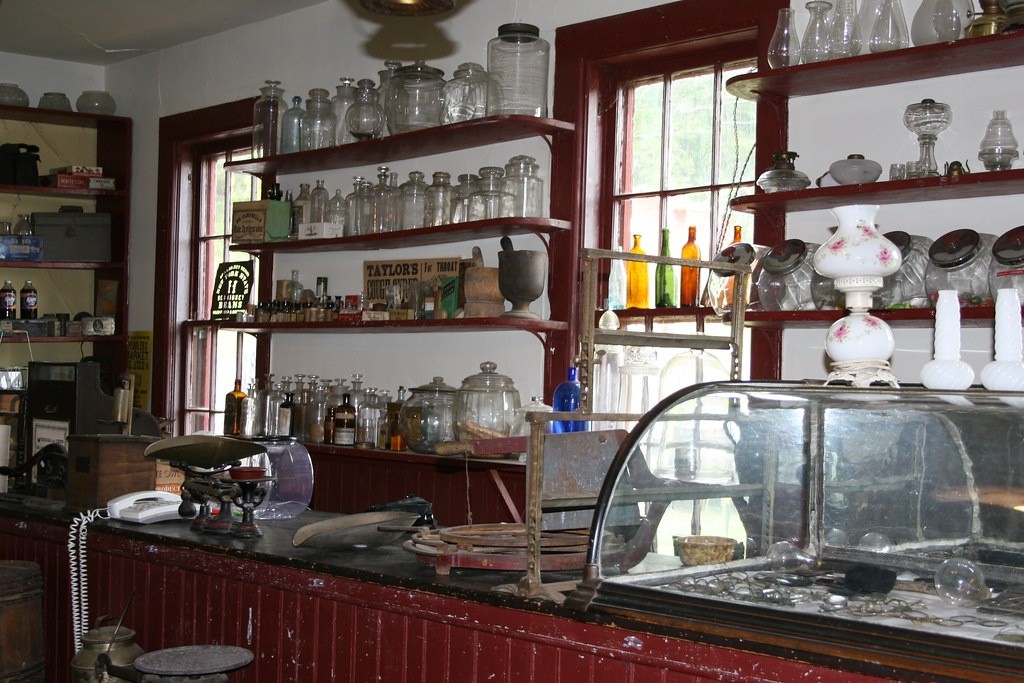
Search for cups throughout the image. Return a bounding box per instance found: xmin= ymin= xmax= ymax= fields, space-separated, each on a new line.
xmin=906 ymin=160 xmax=919 ymax=180
xmin=888 ymin=163 xmax=906 ymax=181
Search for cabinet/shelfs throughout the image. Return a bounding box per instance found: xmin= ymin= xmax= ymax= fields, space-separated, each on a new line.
xmin=219 ymin=113 xmax=581 ymax=474
xmin=722 ymin=31 xmax=1023 ymax=329
xmin=0 ymin=103 xmax=133 ymax=343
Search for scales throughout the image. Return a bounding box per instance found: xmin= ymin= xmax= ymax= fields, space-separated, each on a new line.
xmin=143 ymin=433 xmax=278 ymax=538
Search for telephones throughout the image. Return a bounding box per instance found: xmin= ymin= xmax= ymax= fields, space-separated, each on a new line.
xmin=107 ymin=490 xmax=201 ymax=525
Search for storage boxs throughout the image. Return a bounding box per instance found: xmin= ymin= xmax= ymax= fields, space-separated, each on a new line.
xmin=65 ymin=434 xmax=161 ymax=513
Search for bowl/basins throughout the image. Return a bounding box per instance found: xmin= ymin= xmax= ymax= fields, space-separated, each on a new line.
xmin=674 ymin=535 xmax=735 ymax=567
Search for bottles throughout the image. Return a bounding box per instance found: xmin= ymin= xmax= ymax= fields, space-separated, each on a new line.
xmin=222 ymin=22 xmax=549 ymax=456
xmin=920 ymin=289 xmax=974 ymax=391
xmin=681 ymin=226 xmax=699 ymax=308
xmin=708 ymin=98 xmax=1024 ymax=361
xmin=626 ymin=235 xmax=650 ymax=309
xmin=0 ymin=222 xmax=11 ymax=236
xmin=608 ymin=246 xmax=627 ymax=311
xmin=20 ymin=280 xmax=38 ymax=320
xmin=250 ymin=433 xmax=315 ymax=520
xmin=654 ymin=229 xmax=676 ymax=308
xmin=766 ymin=0 xmax=962 ymax=68
xmin=979 ymin=287 xmax=1024 ymax=394
xmin=553 ymin=367 xmax=588 ymax=434
xmin=13 ymin=213 xmax=32 ymax=235
xmin=726 ymin=226 xmax=749 ymax=306
xmin=0 ymin=281 xmax=17 ymax=320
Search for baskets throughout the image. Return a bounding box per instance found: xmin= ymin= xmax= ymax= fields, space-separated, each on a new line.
xmin=675 ymin=537 xmax=737 ymax=567
